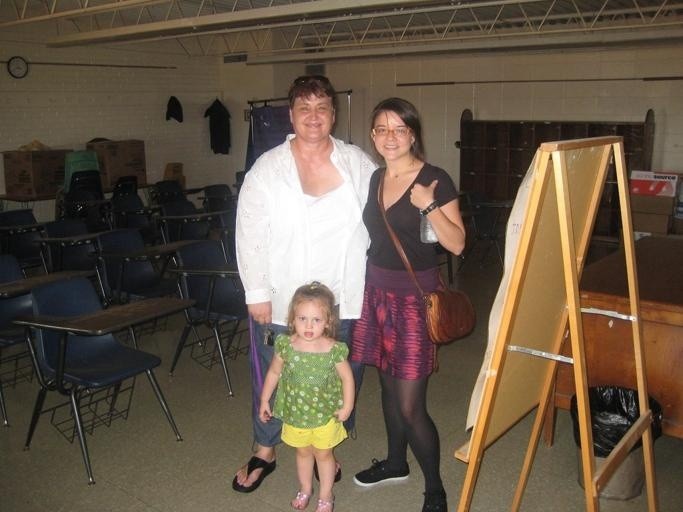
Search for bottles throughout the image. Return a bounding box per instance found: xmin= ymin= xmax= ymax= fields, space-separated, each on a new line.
xmin=418 ymin=200 xmax=438 ymax=242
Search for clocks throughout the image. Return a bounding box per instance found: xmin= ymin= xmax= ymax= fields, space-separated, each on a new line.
xmin=7 ymin=56 xmax=28 ymax=78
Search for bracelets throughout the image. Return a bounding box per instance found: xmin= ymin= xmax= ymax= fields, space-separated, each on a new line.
xmin=418 ymin=200 xmax=439 ymax=218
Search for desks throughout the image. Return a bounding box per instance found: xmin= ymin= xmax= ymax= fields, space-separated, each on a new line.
xmin=543 ymin=234 xmax=683 ymax=448
xmin=0 ymin=184 xmax=155 ymax=212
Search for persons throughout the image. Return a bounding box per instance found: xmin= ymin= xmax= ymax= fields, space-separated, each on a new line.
xmin=258 ymin=281 xmax=356 ymax=512
xmin=352 ymin=97 xmax=467 ymax=512
xmin=232 ymin=74 xmax=380 ymax=493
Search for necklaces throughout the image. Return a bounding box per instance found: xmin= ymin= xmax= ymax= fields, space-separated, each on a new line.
xmin=394 ymin=157 xmax=415 ymax=180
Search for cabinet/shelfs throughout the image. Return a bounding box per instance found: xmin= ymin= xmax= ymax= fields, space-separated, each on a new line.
xmin=453 ymin=107 xmax=656 ymax=247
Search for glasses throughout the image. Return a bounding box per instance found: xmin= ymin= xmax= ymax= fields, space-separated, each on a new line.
xmin=371 ymin=128 xmax=411 ymax=137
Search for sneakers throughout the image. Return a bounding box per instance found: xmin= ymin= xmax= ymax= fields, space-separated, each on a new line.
xmin=353 ymin=460 xmax=410 ymax=487
xmin=422 ymin=488 xmax=447 ymax=512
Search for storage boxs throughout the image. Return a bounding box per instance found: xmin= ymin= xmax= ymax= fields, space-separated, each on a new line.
xmin=631 ymin=170 xmax=682 ymax=198
xmin=631 ymin=212 xmax=674 ymax=236
xmin=0 ymin=149 xmax=74 ymax=197
xmin=85 ymin=138 xmax=148 ymax=189
xmin=629 ymin=193 xmax=675 ymax=215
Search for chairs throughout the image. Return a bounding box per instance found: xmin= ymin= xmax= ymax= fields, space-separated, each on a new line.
xmin=0 ymin=169 xmax=249 ymax=398
xmin=12 ymin=279 xmax=198 ymax=485
xmin=433 ymin=190 xmax=509 ymax=284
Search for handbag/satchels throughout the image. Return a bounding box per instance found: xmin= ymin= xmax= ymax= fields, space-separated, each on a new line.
xmin=423 ymin=288 xmax=475 ymax=345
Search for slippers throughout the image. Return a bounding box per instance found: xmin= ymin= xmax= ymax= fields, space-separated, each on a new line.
xmin=315 ymin=495 xmax=335 ymax=512
xmin=291 ymin=488 xmax=314 ymax=511
xmin=232 ymin=456 xmax=276 ymax=493
xmin=313 ymin=460 xmax=341 ymax=482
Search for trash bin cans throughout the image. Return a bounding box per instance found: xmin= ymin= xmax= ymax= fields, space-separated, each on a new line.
xmin=568 ymin=385 xmax=662 ymax=501
